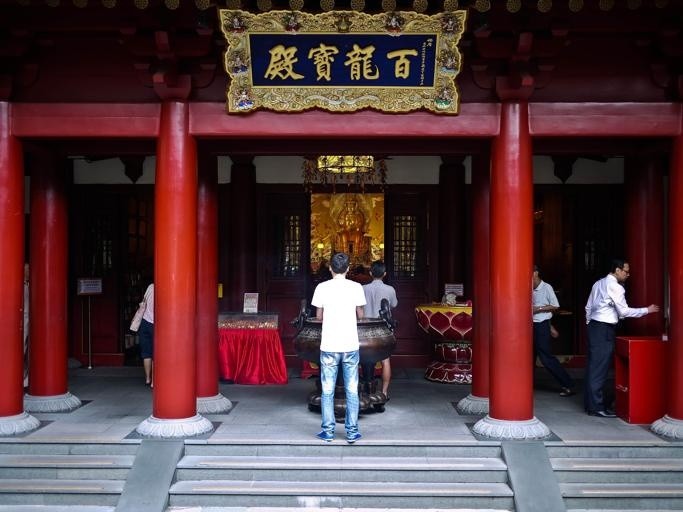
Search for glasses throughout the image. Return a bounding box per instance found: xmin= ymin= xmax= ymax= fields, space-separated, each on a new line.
xmin=619 ymin=268 xmax=630 ymax=275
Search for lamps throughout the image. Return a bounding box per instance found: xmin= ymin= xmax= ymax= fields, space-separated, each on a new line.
xmin=318 ymin=240 xmax=324 ymax=258
xmin=301 ymin=155 xmax=388 ymax=194
xmin=380 ymin=239 xmax=384 ymax=259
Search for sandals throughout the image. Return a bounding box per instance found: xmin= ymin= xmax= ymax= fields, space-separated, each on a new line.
xmin=560 ymin=387 xmax=576 ymax=397
xmin=386 ymin=393 xmax=390 ymax=401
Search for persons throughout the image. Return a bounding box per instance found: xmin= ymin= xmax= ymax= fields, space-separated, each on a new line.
xmin=337 ymin=195 xmax=365 ymax=257
xmin=532 ymin=264 xmax=577 ymax=397
xmin=361 ymin=258 xmax=399 ymax=401
xmin=139 ymin=284 xmax=156 ymax=388
xmin=583 ymin=259 xmax=660 ymax=418
xmin=310 ymin=252 xmax=368 ymax=443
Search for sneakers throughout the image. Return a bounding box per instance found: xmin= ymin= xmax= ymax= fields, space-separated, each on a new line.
xmin=315 ymin=431 xmax=334 ymax=441
xmin=347 ymin=433 xmax=362 ymax=443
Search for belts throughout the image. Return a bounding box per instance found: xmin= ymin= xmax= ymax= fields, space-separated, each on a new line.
xmin=533 ymin=320 xmax=549 ymax=325
xmin=590 ymin=319 xmax=616 ymax=328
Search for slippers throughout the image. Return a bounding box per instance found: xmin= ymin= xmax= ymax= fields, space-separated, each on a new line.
xmin=145 ymin=377 xmax=154 ymax=389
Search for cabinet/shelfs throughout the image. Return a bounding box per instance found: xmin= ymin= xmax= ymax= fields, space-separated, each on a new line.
xmin=615 ymin=334 xmax=669 ymax=425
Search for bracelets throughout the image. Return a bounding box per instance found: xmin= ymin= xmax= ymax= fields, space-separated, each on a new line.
xmin=538 ymin=306 xmax=542 ymax=312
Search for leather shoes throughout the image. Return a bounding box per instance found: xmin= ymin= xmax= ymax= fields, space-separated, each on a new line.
xmin=588 ymin=408 xmax=617 ymax=418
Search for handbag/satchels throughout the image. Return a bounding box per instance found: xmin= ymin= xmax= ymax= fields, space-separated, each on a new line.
xmin=130 ymin=302 xmax=146 ymax=332
